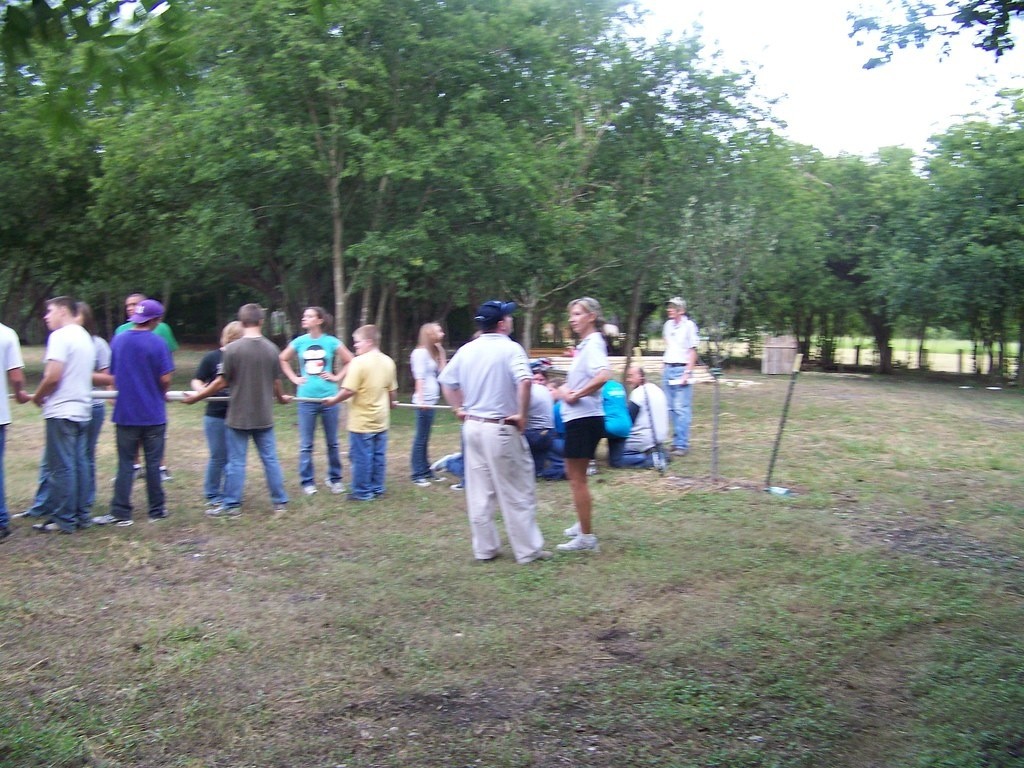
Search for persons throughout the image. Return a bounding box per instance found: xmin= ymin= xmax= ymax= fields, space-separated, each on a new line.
xmin=436 ymin=300 xmax=553 ymax=565
xmin=0 ymin=294 xmax=668 ymax=537
xmin=555 ymin=297 xmax=614 ymax=554
xmin=663 ymin=296 xmax=699 ymax=457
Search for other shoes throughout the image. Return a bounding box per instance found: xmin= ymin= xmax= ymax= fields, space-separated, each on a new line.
xmin=0 ymin=526 xmax=14 ymax=543
xmin=147 ymin=509 xmax=169 ymax=524
xmin=205 ymin=506 xmax=242 ymax=519
xmin=347 ymin=493 xmax=376 ymax=501
xmin=534 ymin=550 xmax=553 ymax=562
xmin=91 ymin=512 xmax=133 ymax=527
xmin=584 ymin=459 xmax=599 ymax=475
xmin=427 ymin=452 xmax=463 ymax=470
xmin=324 ymin=477 xmax=346 ymax=494
xmin=412 ymin=476 xmax=447 ymax=487
xmin=304 ymin=485 xmax=318 ymax=494
xmin=31 ymin=519 xmax=75 ymax=533
xmin=564 ymin=522 xmax=583 ymax=536
xmin=450 ymin=483 xmax=465 ymax=491
xmin=11 ymin=510 xmax=49 ymax=519
xmin=159 ymin=465 xmax=174 ymax=483
xmin=108 ymin=464 xmax=143 ymax=483
xmin=665 ymin=446 xmax=690 ymax=457
xmin=555 ymin=532 xmax=597 ymax=550
xmin=651 ymin=452 xmax=667 ymax=471
xmin=273 ymin=502 xmax=288 ymax=512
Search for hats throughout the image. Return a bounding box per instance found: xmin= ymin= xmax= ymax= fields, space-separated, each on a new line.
xmin=474 ymin=300 xmax=516 ymax=327
xmin=664 ymin=296 xmax=686 ymax=306
xmin=126 ymin=300 xmax=165 ymax=323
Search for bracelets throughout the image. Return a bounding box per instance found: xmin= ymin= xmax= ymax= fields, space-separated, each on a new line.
xmin=684 ymin=370 xmax=692 ymax=374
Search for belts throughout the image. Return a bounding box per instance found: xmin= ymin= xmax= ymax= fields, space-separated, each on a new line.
xmin=664 ymin=362 xmax=688 ymax=368
xmin=464 ymin=415 xmax=517 ymax=425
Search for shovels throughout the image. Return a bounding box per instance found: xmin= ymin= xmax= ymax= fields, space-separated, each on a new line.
xmin=640 ymin=369 xmax=667 ymax=470
